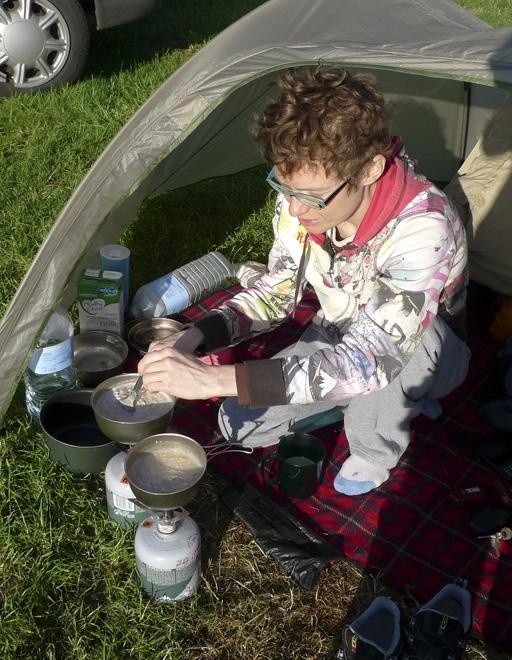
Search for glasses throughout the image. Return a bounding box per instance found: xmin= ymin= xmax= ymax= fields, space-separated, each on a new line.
xmin=265 ymin=158 xmax=363 ymax=212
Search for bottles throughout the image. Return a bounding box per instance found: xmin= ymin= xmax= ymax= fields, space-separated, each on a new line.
xmin=127 ymin=251 xmax=236 ymax=322
xmin=21 ymin=300 xmax=78 ymax=421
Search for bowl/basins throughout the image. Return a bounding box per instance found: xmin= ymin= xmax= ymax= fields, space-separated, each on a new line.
xmin=128 ymin=317 xmax=184 ymax=358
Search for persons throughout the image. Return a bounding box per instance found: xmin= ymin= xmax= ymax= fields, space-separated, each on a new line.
xmin=138 ymin=58 xmax=472 ymax=497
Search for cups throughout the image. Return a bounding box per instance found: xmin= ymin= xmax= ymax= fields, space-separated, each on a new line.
xmin=259 ymin=432 xmax=327 ymax=498
xmin=100 ymin=242 xmax=130 ymax=303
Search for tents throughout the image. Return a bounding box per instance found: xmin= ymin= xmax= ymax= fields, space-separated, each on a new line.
xmin=0 ymin=0 xmax=512 ymax=431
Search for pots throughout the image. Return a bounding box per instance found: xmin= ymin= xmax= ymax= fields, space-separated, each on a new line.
xmin=39 ymin=388 xmax=127 ymax=477
xmin=72 ymin=330 xmax=130 ymax=388
xmin=124 ymin=430 xmax=255 ymax=510
xmin=90 ymin=372 xmax=177 ymax=445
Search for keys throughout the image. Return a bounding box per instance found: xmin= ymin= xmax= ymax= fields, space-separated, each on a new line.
xmin=476 ymin=527 xmax=512 ymax=541
xmin=491 ymin=537 xmax=500 ymax=558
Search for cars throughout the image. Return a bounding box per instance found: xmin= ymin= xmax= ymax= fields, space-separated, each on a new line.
xmin=0 ymin=1 xmax=158 ymax=102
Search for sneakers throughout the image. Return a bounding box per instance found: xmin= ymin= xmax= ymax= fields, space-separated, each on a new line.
xmin=398 ymin=576 xmax=473 ymax=659
xmin=334 ymin=594 xmax=410 ymax=660
